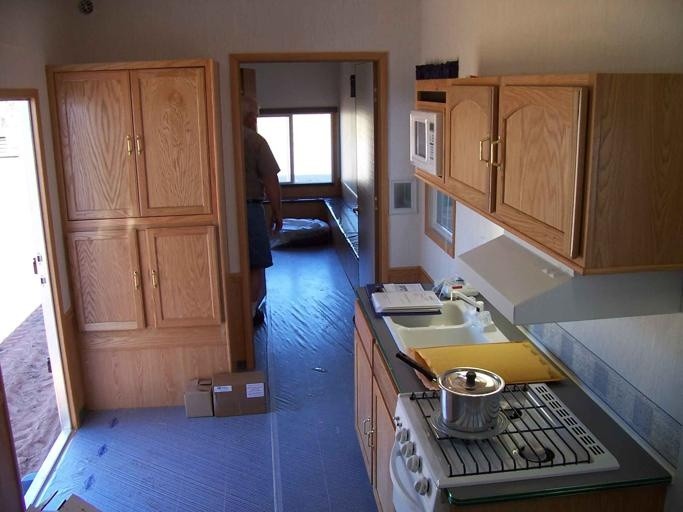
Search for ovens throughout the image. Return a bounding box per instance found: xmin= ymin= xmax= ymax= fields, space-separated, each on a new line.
xmin=384 ymin=442 xmax=454 ymax=512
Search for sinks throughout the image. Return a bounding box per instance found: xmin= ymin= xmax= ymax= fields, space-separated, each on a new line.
xmin=382 ymin=298 xmax=473 ymax=333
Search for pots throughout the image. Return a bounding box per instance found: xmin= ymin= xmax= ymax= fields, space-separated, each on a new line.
xmin=395 ymin=350 xmax=505 ymax=433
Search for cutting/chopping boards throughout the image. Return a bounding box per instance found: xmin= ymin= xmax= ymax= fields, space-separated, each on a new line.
xmin=414 ymin=339 xmax=565 ymax=384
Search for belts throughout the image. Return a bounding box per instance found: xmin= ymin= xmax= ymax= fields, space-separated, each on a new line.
xmin=247 ymin=198 xmax=264 ymax=204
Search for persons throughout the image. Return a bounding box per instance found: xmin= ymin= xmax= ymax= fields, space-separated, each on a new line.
xmin=240 ymin=94 xmax=282 ymax=327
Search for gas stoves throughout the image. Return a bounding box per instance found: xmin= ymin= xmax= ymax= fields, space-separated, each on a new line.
xmin=386 ymin=383 xmax=620 ymax=498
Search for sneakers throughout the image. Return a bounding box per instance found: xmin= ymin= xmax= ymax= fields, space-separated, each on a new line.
xmin=253 ymin=308 xmax=264 ymax=328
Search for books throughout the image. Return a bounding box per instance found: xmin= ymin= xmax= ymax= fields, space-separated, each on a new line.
xmin=363 ymin=282 xmax=444 ymax=318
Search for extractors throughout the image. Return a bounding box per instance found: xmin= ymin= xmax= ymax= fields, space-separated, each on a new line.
xmin=451 ymin=229 xmax=682 ymax=326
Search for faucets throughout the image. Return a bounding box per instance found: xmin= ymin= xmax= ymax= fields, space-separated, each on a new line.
xmin=450 ymin=291 xmax=484 ymax=312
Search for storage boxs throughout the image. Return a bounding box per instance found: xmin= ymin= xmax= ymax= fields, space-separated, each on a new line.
xmin=26 ymin=489 xmax=101 ymax=512
xmin=212 ymin=371 xmax=268 ymax=417
xmin=183 ymin=377 xmax=213 ymax=417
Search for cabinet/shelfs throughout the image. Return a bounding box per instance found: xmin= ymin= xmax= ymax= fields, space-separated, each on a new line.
xmin=414 ymin=74 xmax=683 ymax=276
xmin=47 ymin=58 xmax=246 ymax=412
xmin=352 ymin=298 xmax=403 ymax=512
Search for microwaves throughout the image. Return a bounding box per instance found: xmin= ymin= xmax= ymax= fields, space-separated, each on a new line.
xmin=407 ymin=110 xmax=442 ymax=176
